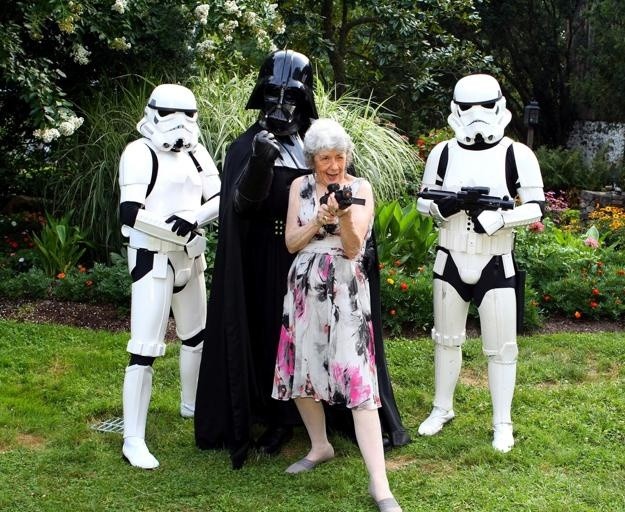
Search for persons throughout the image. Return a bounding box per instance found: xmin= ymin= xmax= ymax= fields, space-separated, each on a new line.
xmin=418 ymin=74 xmax=546 ymax=454
xmin=271 ymin=118 xmax=402 ymax=512
xmin=193 ymin=50 xmax=410 ymax=468
xmin=116 ymin=83 xmax=226 ymax=471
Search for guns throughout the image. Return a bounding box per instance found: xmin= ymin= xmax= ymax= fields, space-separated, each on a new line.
xmin=320 ymin=183 xmax=366 ymax=232
xmin=417 ymin=185 xmax=514 ymax=232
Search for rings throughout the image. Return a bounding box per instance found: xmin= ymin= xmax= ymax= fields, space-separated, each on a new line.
xmin=321 ymin=216 xmax=328 ymax=224
xmin=331 ymin=208 xmax=338 ymax=213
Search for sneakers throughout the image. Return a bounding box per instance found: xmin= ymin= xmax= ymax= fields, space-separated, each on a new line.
xmin=367 ymin=476 xmax=403 ymax=512
xmin=285 ymin=443 xmax=335 ymax=474
